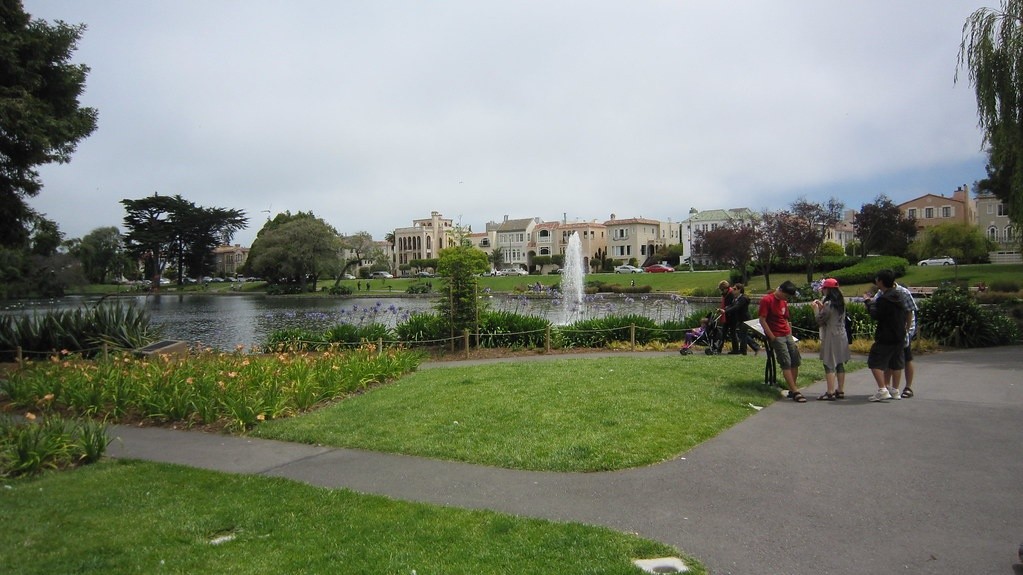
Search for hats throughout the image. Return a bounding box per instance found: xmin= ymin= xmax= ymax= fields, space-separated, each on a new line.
xmin=821 ymin=279 xmax=839 ymax=288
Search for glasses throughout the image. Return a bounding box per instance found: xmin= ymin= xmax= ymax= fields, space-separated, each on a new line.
xmin=721 ymin=288 xmax=725 ymax=291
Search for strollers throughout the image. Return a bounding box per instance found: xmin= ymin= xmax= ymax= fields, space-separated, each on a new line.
xmin=680 ymin=307 xmax=723 ymax=356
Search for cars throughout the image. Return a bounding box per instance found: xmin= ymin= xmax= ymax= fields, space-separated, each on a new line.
xmin=917 ymin=255 xmax=955 ymax=265
xmin=614 ymin=266 xmax=643 ymax=274
xmin=143 ymin=276 xmax=236 ymax=282
xmin=343 ymin=274 xmax=356 ymax=280
xmin=373 ymin=272 xmax=393 ymax=279
xmin=414 ymin=272 xmax=435 ymax=278
xmin=477 ymin=268 xmax=528 ymax=276
xmin=646 ymin=264 xmax=674 ymax=273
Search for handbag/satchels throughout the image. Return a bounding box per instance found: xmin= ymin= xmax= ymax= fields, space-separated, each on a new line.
xmin=845 ymin=308 xmax=853 ymax=344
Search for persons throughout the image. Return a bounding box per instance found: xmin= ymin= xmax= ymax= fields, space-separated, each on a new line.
xmin=365 ymin=281 xmax=370 ymax=291
xmin=357 ymin=281 xmax=361 ymax=290
xmin=681 ymin=317 xmax=709 ymax=348
xmin=717 ymin=280 xmax=760 ymax=355
xmin=811 ymin=278 xmax=849 ymax=400
xmin=758 ymin=281 xmax=807 ymax=402
xmin=863 ymin=270 xmax=920 ymax=402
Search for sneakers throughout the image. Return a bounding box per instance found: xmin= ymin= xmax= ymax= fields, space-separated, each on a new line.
xmin=889 ymin=389 xmax=901 ymax=399
xmin=868 ymin=389 xmax=891 ymax=401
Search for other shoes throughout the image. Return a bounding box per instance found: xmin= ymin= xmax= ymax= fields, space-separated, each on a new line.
xmin=728 ymin=351 xmax=738 ymax=354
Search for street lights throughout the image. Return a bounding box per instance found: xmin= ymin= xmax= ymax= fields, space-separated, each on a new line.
xmin=689 ymin=207 xmax=698 ymax=272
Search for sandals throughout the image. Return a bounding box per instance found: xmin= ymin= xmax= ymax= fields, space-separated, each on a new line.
xmin=817 ymin=392 xmax=836 ymax=400
xmin=835 ymin=390 xmax=844 ymax=398
xmin=901 ymin=388 xmax=913 ymax=398
xmin=793 ymin=392 xmax=806 ymax=402
xmin=787 ymin=391 xmax=793 ymax=397
